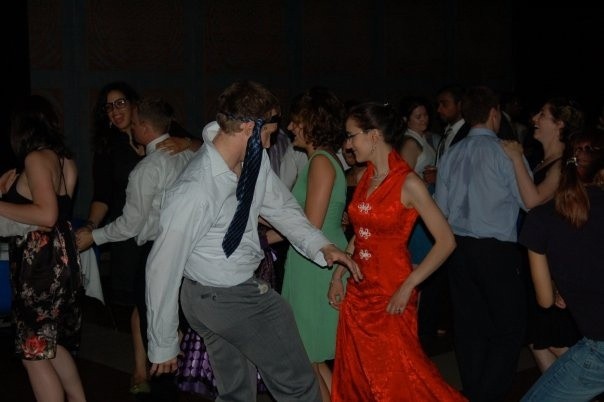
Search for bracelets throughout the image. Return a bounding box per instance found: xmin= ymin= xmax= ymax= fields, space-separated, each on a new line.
xmin=183 ymin=136 xmax=192 ymax=149
xmin=81 ymin=220 xmax=98 ymax=232
xmin=331 ymin=279 xmax=342 ymax=284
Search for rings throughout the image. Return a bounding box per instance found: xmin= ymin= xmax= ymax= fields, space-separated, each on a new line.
xmin=328 ymin=301 xmax=333 ymax=305
xmin=395 ymin=308 xmax=400 ymax=312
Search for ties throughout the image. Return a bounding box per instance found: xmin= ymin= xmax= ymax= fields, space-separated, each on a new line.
xmin=222 ymin=113 xmax=281 ymax=258
xmin=438 ymin=128 xmax=454 ymax=160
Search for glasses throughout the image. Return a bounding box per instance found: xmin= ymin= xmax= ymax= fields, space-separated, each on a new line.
xmin=346 ymin=130 xmax=367 ymax=141
xmin=105 ymin=98 xmax=130 ymax=112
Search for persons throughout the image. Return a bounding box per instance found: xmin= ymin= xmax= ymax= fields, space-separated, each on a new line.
xmin=72 ymin=96 xmax=196 ymax=378
xmin=517 ymin=128 xmax=604 ymax=402
xmin=171 ymin=215 xmax=276 ymax=402
xmin=397 ymin=97 xmax=441 ymax=190
xmin=257 ymin=87 xmax=350 ymax=402
xmin=495 ymin=91 xmax=524 ymax=143
xmin=496 ymin=97 xmax=583 ymax=373
xmin=0 ymin=95 xmax=86 ymax=402
xmin=328 ymin=102 xmax=456 ymax=402
xmin=74 ymin=80 xmax=204 ymax=394
xmin=264 ymin=123 xmax=299 ymax=294
xmin=431 ymin=85 xmax=535 ymax=402
xmin=339 ymin=135 xmax=367 ymax=242
xmin=422 ymin=86 xmax=469 ymax=185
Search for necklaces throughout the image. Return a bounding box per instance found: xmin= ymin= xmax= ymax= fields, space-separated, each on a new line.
xmin=533 ymin=152 xmax=562 ymax=172
xmin=369 ymin=168 xmax=388 ymax=189
